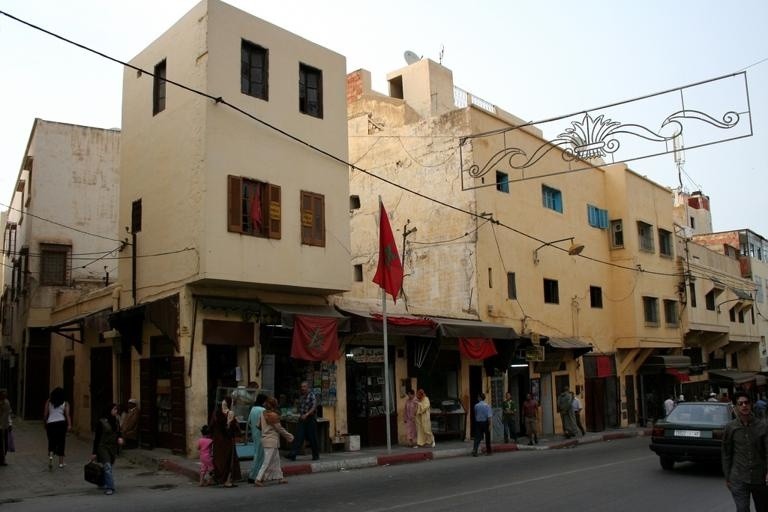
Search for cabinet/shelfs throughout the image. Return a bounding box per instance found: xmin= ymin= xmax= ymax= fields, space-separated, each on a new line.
xmin=214 ymin=386 xmax=273 ymax=431
xmin=352 ymin=363 xmax=394 ymax=446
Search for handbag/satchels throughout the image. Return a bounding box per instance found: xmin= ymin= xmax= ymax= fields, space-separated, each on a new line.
xmin=84 ymin=462 xmax=106 ymax=486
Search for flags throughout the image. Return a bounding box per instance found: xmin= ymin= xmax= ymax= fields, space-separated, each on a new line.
xmin=290 ymin=316 xmax=339 ymax=361
xmin=370 ymin=203 xmax=403 ymax=303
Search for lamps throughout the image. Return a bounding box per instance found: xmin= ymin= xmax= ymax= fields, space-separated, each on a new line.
xmin=534 ymin=229 xmax=585 ymax=258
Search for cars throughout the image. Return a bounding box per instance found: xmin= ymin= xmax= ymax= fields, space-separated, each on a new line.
xmin=649 ymin=401 xmax=738 ymax=476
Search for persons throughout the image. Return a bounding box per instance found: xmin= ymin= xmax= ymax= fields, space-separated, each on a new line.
xmin=414 ymin=387 xmax=436 ymax=449
xmin=196 ymin=424 xmax=216 ymax=486
xmin=118 ymin=397 xmax=140 ymax=441
xmin=253 ymin=396 xmax=294 ymax=485
xmin=90 ymin=402 xmax=123 ymax=495
xmin=403 ymin=389 xmax=418 ymax=447
xmin=717 ymin=393 xmax=768 ymax=511
xmin=556 ymin=385 xmax=577 ymax=435
xmin=520 ymin=393 xmax=540 ymax=444
xmin=248 ymin=394 xmax=267 ymax=484
xmin=501 ymin=391 xmax=517 ymax=443
xmin=680 ymin=391 xmax=766 ymax=418
xmin=209 ymin=394 xmax=242 ymax=487
xmin=230 ymin=380 xmax=260 ymax=420
xmin=471 ymin=392 xmax=492 ymax=454
xmin=284 ymin=379 xmax=322 ymax=460
xmin=43 ymin=387 xmax=71 ymax=471
xmin=663 ymin=393 xmax=675 ymax=414
xmin=569 ymin=392 xmax=585 ymax=435
xmin=1 ymin=386 xmax=14 ymax=468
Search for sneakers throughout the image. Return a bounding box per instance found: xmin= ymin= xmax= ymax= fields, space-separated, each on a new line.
xmin=58 ymin=463 xmax=67 ymax=468
xmin=283 ymin=452 xmax=296 ymax=461
xmin=223 ymin=483 xmax=239 ymax=488
xmin=413 ymin=445 xmax=419 ymax=448
xmin=198 ymin=479 xmax=218 ymax=487
xmin=312 ymin=455 xmax=319 ymax=460
xmin=279 ymin=479 xmax=288 ymax=484
xmin=48 ymin=457 xmax=53 ymax=471
xmin=431 ymin=437 xmax=436 ymax=448
xmin=248 ymin=478 xmax=266 ymax=487
xmin=104 ymin=488 xmax=113 ymax=495
